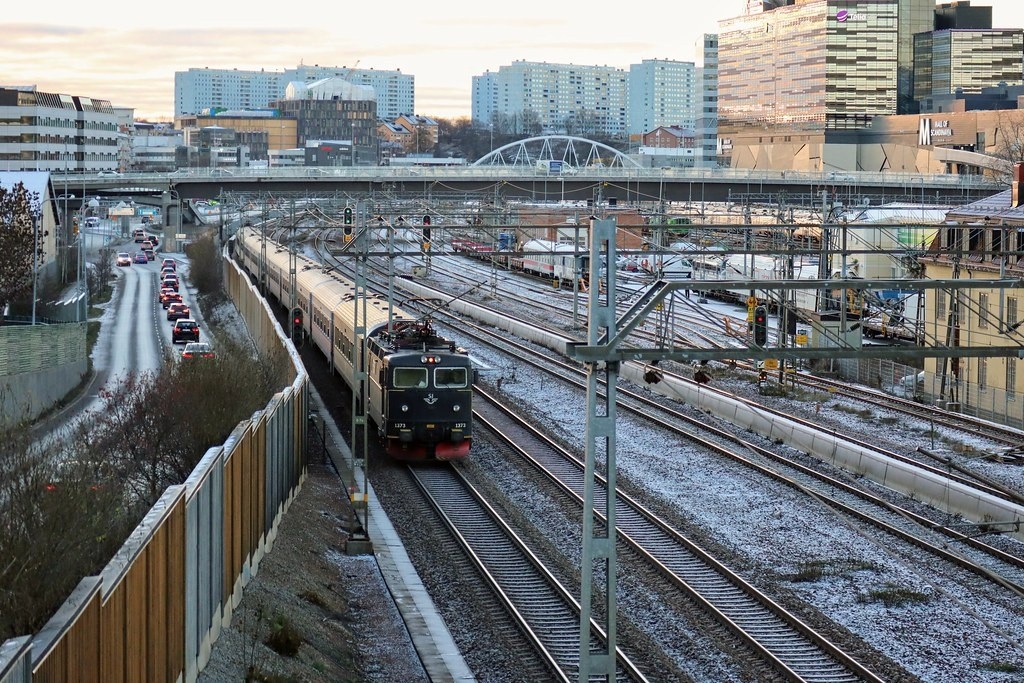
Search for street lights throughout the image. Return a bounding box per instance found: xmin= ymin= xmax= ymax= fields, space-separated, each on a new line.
xmin=163 ymin=189 xmax=179 ymax=252
xmin=32 ymin=196 xmax=100 ymax=327
xmin=76 ymin=198 xmax=100 ymax=321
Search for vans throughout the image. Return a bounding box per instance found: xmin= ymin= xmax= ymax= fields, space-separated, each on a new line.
xmin=638 ymin=253 xmax=693 ymax=273
xmin=195 ymin=201 xmax=210 ymax=206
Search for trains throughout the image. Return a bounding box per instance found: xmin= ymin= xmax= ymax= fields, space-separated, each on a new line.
xmin=641 ymin=216 xmax=691 ymax=235
xmin=688 ymin=255 xmax=864 ymax=321
xmin=236 ymin=226 xmax=478 ymax=465
xmin=452 ymin=235 xmax=595 ymax=286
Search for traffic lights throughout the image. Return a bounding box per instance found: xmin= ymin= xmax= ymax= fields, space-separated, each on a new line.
xmin=423 ymin=215 xmax=430 ymax=243
xmin=344 ymin=208 xmax=353 ymax=234
xmin=754 ymin=306 xmax=767 ymax=347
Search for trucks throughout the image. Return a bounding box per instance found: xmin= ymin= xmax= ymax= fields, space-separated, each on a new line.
xmin=537 ymin=161 xmax=577 ymax=175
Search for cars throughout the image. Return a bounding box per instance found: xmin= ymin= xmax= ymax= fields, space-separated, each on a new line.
xmin=146 ymin=236 xmax=159 ymax=245
xmin=141 ymin=241 xmax=153 ymax=250
xmin=303 ymin=168 xmax=330 ymax=178
xmin=163 ymin=274 xmax=178 ymax=284
xmin=132 ymin=252 xmax=148 ymax=264
xmin=85 ymin=217 xmax=100 ymax=227
xmin=144 ymin=250 xmax=155 ymax=261
xmin=165 ymin=302 xmax=190 ymax=320
xmin=179 ymin=343 xmax=216 ymax=364
xmin=158 ymin=287 xmax=175 ymax=302
xmin=161 ymin=292 xmax=183 ymax=308
xmin=598 ymin=252 xmax=638 ymax=271
xmin=116 ymin=253 xmax=131 ymax=267
xmin=39 ymin=458 xmax=123 ymax=512
xmin=160 ymin=267 xmax=176 ymax=279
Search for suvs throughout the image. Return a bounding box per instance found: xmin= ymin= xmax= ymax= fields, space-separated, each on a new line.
xmin=210 ymin=169 xmax=233 ymax=176
xmin=168 ymin=170 xmax=195 ymax=177
xmin=135 ymin=232 xmax=146 ymax=242
xmin=392 ymin=168 xmax=420 ymax=177
xmin=170 ymin=318 xmax=200 ymax=343
xmin=161 ymin=259 xmax=177 ymax=270
xmin=141 ymin=217 xmax=153 ymax=224
xmin=160 ymin=279 xmax=179 ymax=292
xmin=133 ymin=228 xmax=144 ymax=236
xmin=97 ymin=171 xmax=124 ymax=178
